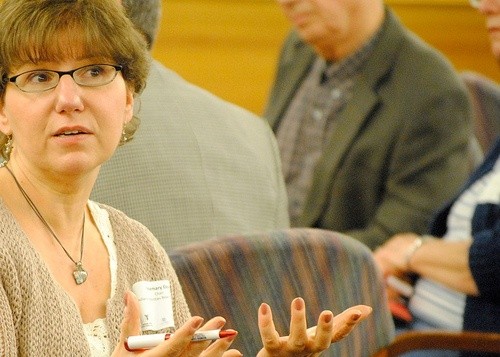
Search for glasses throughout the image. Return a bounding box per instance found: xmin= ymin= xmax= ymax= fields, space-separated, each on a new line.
xmin=2 ymin=63 xmax=124 ymax=93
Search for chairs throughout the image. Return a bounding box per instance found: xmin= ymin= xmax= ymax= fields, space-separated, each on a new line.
xmin=163 ymin=229 xmax=500 ymax=357
xmin=457 ymin=71 xmax=500 ymax=161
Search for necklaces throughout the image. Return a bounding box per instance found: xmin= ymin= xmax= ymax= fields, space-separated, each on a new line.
xmin=4 ymin=163 xmax=88 ymax=286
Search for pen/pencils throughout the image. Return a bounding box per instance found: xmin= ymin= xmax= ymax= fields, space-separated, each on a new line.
xmin=123 ymin=329 xmax=237 ymax=352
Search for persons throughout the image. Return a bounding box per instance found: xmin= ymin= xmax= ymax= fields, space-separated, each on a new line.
xmin=0 ymin=0 xmax=372 ymax=357
xmin=373 ymin=137 xmax=499 ymax=357
xmin=263 ymin=0 xmax=484 ymax=327
xmin=88 ymin=0 xmax=289 ymax=255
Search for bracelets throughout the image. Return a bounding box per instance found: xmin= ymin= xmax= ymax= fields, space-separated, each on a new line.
xmin=405 ymin=233 xmax=435 ymax=275
